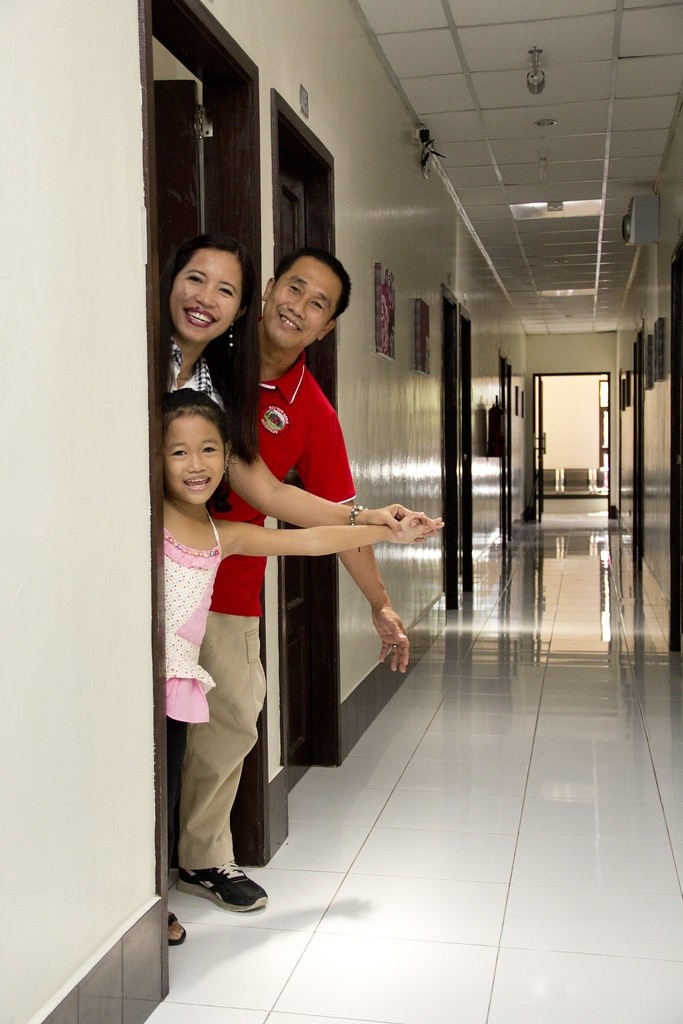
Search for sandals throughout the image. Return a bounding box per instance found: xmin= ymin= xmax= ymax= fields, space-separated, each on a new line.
xmin=167 ymin=913 xmax=186 ymax=946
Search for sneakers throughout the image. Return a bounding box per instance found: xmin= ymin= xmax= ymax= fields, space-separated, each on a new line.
xmin=176 ymin=862 xmax=268 ymax=912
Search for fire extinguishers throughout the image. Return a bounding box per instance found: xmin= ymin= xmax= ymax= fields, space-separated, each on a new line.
xmin=486 ymin=395 xmax=506 ymax=458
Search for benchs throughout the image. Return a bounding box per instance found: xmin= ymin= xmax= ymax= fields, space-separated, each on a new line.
xmin=536 ymin=467 xmax=609 ymax=498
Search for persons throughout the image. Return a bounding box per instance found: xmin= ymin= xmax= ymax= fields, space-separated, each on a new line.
xmin=153 ymin=237 xmax=446 ymax=954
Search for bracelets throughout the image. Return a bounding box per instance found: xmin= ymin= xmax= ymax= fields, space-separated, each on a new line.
xmin=349 ymin=505 xmax=368 ymax=526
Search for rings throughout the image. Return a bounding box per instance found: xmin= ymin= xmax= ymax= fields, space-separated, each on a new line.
xmin=391 ymin=644 xmax=398 ymax=647
